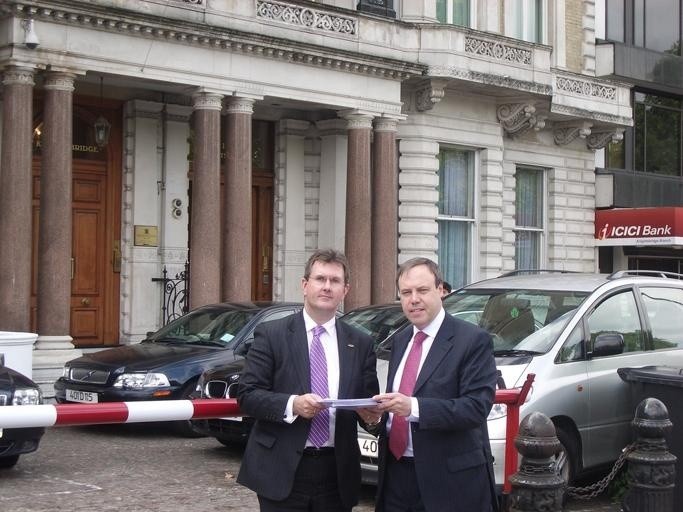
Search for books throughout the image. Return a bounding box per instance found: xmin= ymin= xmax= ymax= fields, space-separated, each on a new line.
xmin=316 ymin=397 xmax=378 ymax=409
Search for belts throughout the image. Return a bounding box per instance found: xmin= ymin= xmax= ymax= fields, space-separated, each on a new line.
xmin=303 ymin=446 xmax=335 ymax=456
xmin=392 ymin=455 xmax=414 ymax=464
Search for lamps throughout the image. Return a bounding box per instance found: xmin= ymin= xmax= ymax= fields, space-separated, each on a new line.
xmin=93 ymin=76 xmax=111 ymax=149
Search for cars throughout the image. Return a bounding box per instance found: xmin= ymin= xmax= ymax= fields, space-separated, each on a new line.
xmin=191 ymin=300 xmax=410 ymax=448
xmin=55 ymin=300 xmax=344 ymax=437
xmin=1 ymin=355 xmax=45 ymax=469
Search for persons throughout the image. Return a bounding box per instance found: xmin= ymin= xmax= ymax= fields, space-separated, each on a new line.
xmin=442 ymin=280 xmax=452 ymax=296
xmin=363 ymin=254 xmax=500 ymax=512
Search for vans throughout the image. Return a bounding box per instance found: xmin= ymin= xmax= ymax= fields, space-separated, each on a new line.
xmin=358 ymin=268 xmax=681 ymax=494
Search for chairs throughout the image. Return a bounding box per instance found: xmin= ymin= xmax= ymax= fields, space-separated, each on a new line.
xmin=491 ymin=299 xmax=580 ymax=361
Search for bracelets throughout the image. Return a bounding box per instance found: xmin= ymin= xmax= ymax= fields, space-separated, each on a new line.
xmin=365 ymin=417 xmax=382 ymax=427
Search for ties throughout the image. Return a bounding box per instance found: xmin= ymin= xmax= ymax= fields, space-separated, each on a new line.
xmin=304 ymin=323 xmax=330 ymax=450
xmin=386 ymin=331 xmax=431 ymax=461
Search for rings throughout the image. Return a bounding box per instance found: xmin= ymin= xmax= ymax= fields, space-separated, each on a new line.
xmin=367 ymin=415 xmax=370 ymax=420
xmin=235 ymin=244 xmax=385 ymax=511
xmin=313 ymin=408 xmax=316 ymax=414
